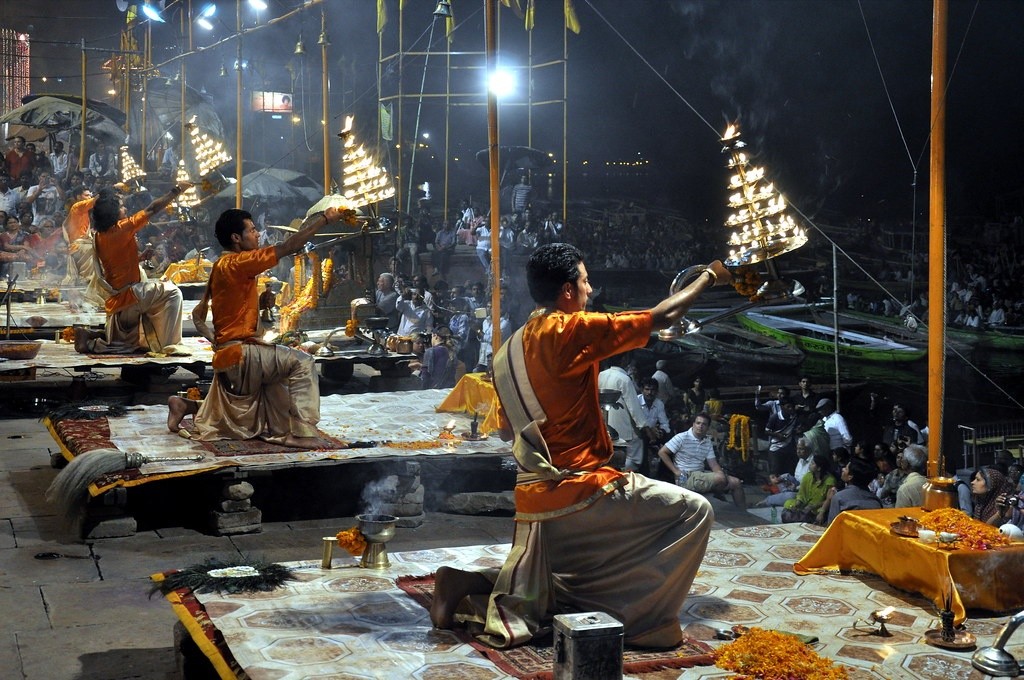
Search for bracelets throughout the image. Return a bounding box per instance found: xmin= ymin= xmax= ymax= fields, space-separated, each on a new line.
xmin=701 ymin=268 xmax=717 ymax=289
xmin=323 ymin=215 xmax=328 ymax=225
xmin=171 ymin=185 xmax=182 ymax=194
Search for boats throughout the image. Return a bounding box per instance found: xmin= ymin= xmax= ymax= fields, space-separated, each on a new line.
xmin=808 ymin=303 xmax=975 ymax=359
xmin=599 ymin=298 xmax=828 ymax=322
xmin=730 ymin=304 xmax=929 ymax=363
xmin=705 ymin=374 xmax=872 ymax=406
xmin=882 ymin=380 xmax=1024 ymax=415
xmin=842 ymin=304 xmax=1024 ymax=354
xmin=643 ymin=320 xmax=806 ymax=371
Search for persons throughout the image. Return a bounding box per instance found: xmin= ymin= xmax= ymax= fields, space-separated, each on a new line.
xmin=396 ymin=198 xmax=730 ymax=290
xmin=755 ymin=376 xmax=1024 ymax=532
xmin=658 ymin=414 xmax=746 ymax=510
xmin=0 ymin=135 xmax=166 ymax=273
xmin=598 ymin=351 xmax=656 ymax=471
xmin=511 ymin=174 xmax=538 ymax=211
xmin=162 ymin=137 xmax=181 ymax=177
xmin=75 ymin=181 xmax=191 ymax=355
xmin=638 ymin=359 xmax=723 ymax=478
xmin=61 ymin=182 xmax=124 ymax=285
xmin=375 ymin=255 xmax=520 ymax=389
xmin=429 ymin=243 xmax=731 ymax=647
xmin=169 ymin=221 xmax=196 ymax=259
xmin=167 ymin=208 xmax=345 ymax=449
xmin=847 ymin=209 xmax=1024 ymax=335
xmin=258 ymin=215 xmax=312 ymax=286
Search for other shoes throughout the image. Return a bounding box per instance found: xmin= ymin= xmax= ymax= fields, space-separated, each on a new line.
xmin=485 ymin=267 xmax=490 ymax=275
xmin=432 ymin=270 xmax=439 ymax=276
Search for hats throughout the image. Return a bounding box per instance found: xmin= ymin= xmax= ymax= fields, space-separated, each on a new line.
xmin=432 ymin=325 xmax=453 ymax=339
xmin=816 ymin=398 xmax=834 ymax=410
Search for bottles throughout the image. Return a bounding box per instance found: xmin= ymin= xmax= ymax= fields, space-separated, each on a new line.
xmin=920 ymin=475 xmax=958 ymax=512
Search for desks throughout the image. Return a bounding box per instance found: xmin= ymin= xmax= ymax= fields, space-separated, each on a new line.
xmin=434 ymin=372 xmax=502 ymax=436
xmin=159 ymin=262 xmax=213 ymax=283
xmin=792 ymin=507 xmax=1024 ymax=627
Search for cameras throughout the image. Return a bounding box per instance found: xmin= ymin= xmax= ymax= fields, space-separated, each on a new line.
xmin=1003 ymin=495 xmax=1019 ymax=507
xmin=44 ymin=177 xmax=50 ymax=184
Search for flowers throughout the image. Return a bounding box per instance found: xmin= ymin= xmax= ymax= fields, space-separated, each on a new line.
xmin=335 ymin=527 xmax=367 ymax=556
xmin=345 ymin=318 xmax=359 ymax=338
xmin=48 ymin=287 xmax=63 ymax=303
xmin=277 ymin=250 xmax=332 ymax=335
xmin=63 ymin=326 xmax=75 ymax=343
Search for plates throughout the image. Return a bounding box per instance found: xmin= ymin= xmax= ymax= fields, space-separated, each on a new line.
xmin=890 ymin=522 xmax=924 ymax=537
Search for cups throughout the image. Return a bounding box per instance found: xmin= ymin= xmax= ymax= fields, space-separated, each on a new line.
xmin=900 ymin=518 xmax=916 ymax=532
